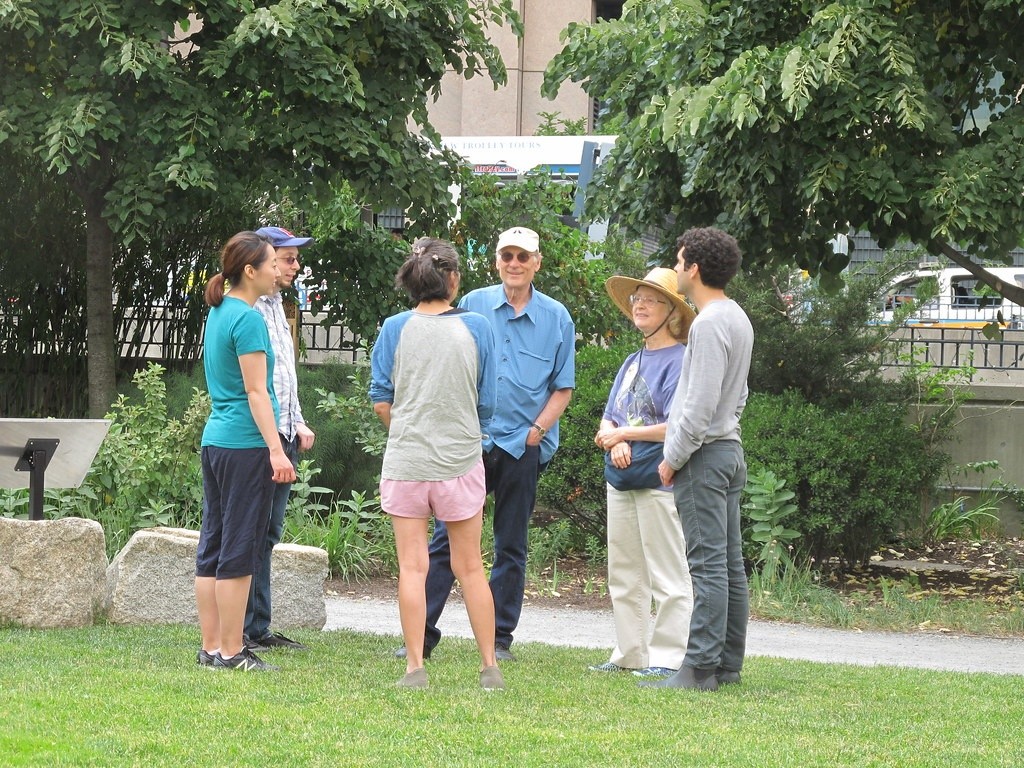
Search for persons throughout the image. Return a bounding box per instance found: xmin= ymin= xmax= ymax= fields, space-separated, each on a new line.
xmin=244 ymin=226 xmax=315 ymax=649
xmin=653 ymin=227 xmax=754 ymax=692
xmin=590 ymin=267 xmax=698 ymax=677
xmin=193 ymin=231 xmax=297 ymax=672
xmin=395 ymin=227 xmax=578 ymax=660
xmin=371 ymin=239 xmax=503 ymax=691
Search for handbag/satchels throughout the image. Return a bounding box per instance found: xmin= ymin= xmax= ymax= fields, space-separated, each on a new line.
xmin=604 ymin=440 xmax=665 ymax=492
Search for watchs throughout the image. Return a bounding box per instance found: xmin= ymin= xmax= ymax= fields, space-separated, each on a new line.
xmin=533 ymin=423 xmax=546 ymax=435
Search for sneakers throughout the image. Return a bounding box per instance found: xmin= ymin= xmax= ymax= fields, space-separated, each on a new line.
xmin=197 ymin=648 xmax=222 ymax=670
xmin=631 ymin=667 xmax=678 ymax=678
xmin=589 ymin=662 xmax=641 ymax=674
xmin=214 ymin=646 xmax=279 ymax=673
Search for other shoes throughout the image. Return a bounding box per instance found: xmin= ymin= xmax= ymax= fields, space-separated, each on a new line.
xmin=242 ymin=634 xmax=270 ymax=652
xmin=255 ymin=632 xmax=307 ymax=650
xmin=495 ymin=643 xmax=516 ymax=663
xmin=718 ymin=671 xmax=740 ymax=686
xmin=480 ymin=665 xmax=504 ymax=692
xmin=397 ymin=642 xmax=431 ymax=659
xmin=637 ymin=669 xmax=717 ymax=693
xmin=388 ymin=666 xmax=429 ymax=691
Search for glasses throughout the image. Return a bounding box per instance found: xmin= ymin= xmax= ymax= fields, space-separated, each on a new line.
xmin=498 ymin=251 xmax=536 ymax=263
xmin=629 ymin=295 xmax=668 ymax=309
xmin=277 ymin=256 xmax=298 ymax=264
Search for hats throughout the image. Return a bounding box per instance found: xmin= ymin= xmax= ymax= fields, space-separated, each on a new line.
xmin=255 ymin=227 xmax=313 ymax=246
xmin=496 ymin=227 xmax=539 ymax=256
xmin=605 ymin=267 xmax=697 ymax=340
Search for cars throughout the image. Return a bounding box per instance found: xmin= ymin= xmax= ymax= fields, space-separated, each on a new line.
xmin=822 ymin=265 xmax=1024 ymax=327
